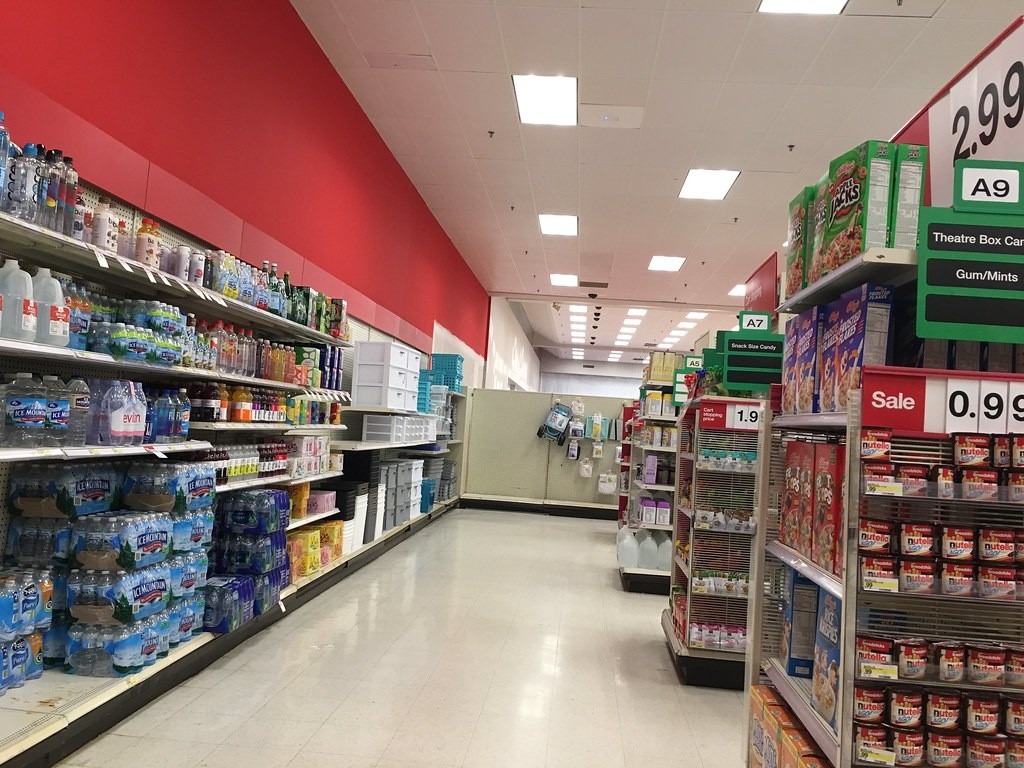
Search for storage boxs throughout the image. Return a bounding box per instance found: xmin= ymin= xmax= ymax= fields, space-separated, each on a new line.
xmin=293 ymin=286 xmax=347 ymax=334
xmin=642 ymin=455 xmax=657 ymax=484
xmin=637 ymin=496 xmax=671 ymax=526
xmin=293 ymin=341 xmax=345 ymax=390
xmin=647 ymin=351 xmax=684 ymax=382
xmin=343 ymin=449 xmax=458 ymax=544
xmin=265 ymin=436 xmax=344 ymax=479
xmin=362 ymin=414 xmax=436 ymax=442
xmin=784 ymin=140 xmax=929 ymax=300
xmin=285 ymin=520 xmax=344 ymax=583
xmin=417 ymin=353 xmax=465 ymax=432
xmin=641 ymin=424 xmax=677 ymax=448
xmin=320 ymin=481 xmax=369 ymax=556
xmin=780 ymin=282 xmax=895 ymax=415
xmin=778 ymin=442 xmax=847 ymax=579
xmin=669 ymin=584 xmax=687 ymax=644
xmin=266 ymin=482 xmax=336 ymax=519
xmin=639 ymin=388 xmax=662 ymax=416
xmin=746 ymin=685 xmax=834 ymax=768
xmin=288 ymin=399 xmax=341 ymax=425
xmin=779 ymin=563 xmax=842 ymax=736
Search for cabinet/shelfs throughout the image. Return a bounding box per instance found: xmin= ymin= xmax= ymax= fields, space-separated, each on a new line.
xmin=745 ymin=247 xmax=1024 ymax=768
xmin=617 ymin=383 xmax=680 ymax=596
xmin=1 ymin=211 xmax=347 ymax=768
xmin=329 ymin=392 xmax=466 ymax=575
xmin=350 ymin=341 xmax=421 ymax=411
xmin=662 ymin=396 xmax=784 ymax=691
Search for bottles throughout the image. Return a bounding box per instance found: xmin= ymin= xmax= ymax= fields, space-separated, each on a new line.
xmin=0 ymin=366 xmax=290 ymax=487
xmin=0 ymin=111 xmax=309 ymax=325
xmin=1 ymin=462 xmax=292 ymax=702
xmin=0 ymin=256 xmax=297 ymax=384
xmin=617 ymin=525 xmax=675 ymax=572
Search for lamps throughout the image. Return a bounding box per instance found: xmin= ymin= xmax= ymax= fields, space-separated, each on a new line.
xmin=553 ymin=302 xmax=561 ymax=311
